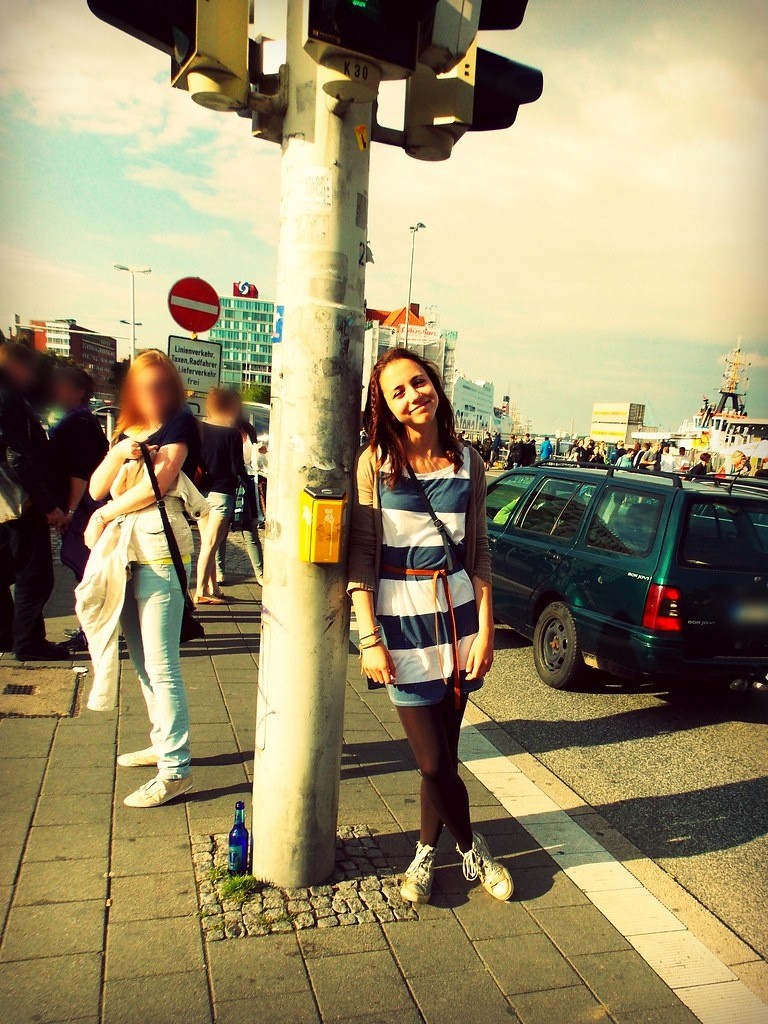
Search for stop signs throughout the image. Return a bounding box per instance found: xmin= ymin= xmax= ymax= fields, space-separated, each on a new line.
xmin=168 ymin=277 xmax=221 ymax=333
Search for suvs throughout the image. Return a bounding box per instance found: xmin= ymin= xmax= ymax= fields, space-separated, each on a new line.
xmin=487 ymin=459 xmax=768 ymax=689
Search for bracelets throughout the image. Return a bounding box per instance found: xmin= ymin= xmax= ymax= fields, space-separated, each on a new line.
xmin=69 ymin=509 xmax=75 ymax=514
xmin=357 ymin=626 xmax=382 ymax=659
xmin=95 ymin=510 xmax=107 ymax=525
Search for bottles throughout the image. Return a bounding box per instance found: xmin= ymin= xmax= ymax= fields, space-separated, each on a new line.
xmin=227 ymin=801 xmax=249 ymax=875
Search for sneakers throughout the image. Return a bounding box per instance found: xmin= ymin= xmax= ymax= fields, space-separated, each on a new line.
xmin=399 ymin=840 xmax=439 ymax=904
xmin=117 ymin=746 xmax=160 ymax=768
xmin=456 ymin=831 xmax=515 ymax=902
xmin=123 ymin=777 xmax=195 ymax=808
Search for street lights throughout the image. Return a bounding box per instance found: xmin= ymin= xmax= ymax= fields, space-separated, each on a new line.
xmin=114 ymin=264 xmax=152 ymax=367
xmin=404 ymin=222 xmax=426 ymax=349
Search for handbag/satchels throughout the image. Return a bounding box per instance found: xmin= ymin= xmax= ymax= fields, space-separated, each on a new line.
xmin=179 ymin=597 xmax=205 ymax=644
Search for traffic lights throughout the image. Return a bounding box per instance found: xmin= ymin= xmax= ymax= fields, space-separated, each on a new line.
xmin=300 ymin=1 xmax=423 ymax=80
xmin=86 ymin=0 xmax=255 ymax=112
xmin=403 ymin=0 xmax=542 ymax=161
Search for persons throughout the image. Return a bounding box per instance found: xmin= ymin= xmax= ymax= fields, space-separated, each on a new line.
xmin=493 ymin=484 xmax=556 ymax=525
xmin=83 ymin=350 xmax=195 ymax=806
xmin=568 ymin=437 xmax=710 ymax=481
xmin=0 ymin=340 xmax=110 ymax=660
xmin=539 ymin=437 xmax=553 ymax=460
xmin=347 ymin=347 xmax=514 ymax=904
xmin=719 ymin=451 xmax=768 ymax=477
xmin=188 ymin=386 xmax=268 ymax=604
xmin=458 ymin=430 xmax=536 ymax=472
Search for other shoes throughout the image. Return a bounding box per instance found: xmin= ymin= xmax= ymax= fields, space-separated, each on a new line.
xmin=14 ymin=641 xmax=72 ymax=662
xmin=59 ymin=631 xmax=88 ymax=651
xmin=212 ymin=592 xmax=224 ymax=598
xmin=192 ymin=594 xmax=228 ymax=605
xmin=61 ymin=628 xmax=81 ymax=637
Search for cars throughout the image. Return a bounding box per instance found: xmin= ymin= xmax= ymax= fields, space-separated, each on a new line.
xmin=44 ymin=393 xmax=270 ymax=484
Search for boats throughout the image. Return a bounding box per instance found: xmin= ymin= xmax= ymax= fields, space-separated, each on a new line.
xmin=676 ymin=336 xmax=768 ymax=477
